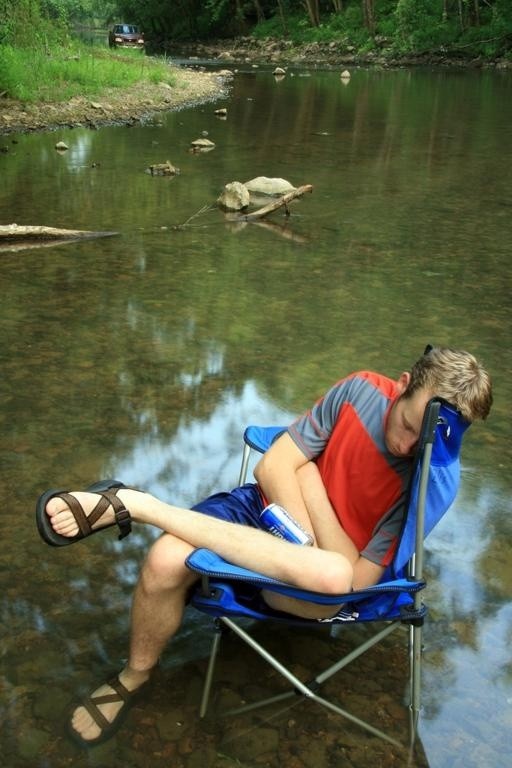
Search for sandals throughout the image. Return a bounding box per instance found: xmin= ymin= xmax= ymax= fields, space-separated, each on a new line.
xmin=36 ymin=479 xmax=132 ymax=547
xmin=66 ymin=668 xmax=157 ymax=748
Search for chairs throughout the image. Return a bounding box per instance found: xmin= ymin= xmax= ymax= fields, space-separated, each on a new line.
xmin=185 ymin=345 xmax=470 ymax=753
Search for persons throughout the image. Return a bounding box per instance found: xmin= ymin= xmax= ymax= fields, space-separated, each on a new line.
xmin=34 ymin=344 xmax=495 ymax=747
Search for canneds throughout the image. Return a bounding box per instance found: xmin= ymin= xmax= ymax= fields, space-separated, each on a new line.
xmin=260 ymin=502 xmax=313 ymax=548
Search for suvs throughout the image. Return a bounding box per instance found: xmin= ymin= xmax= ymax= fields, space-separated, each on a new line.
xmin=109 ymin=23 xmax=146 ymax=54
